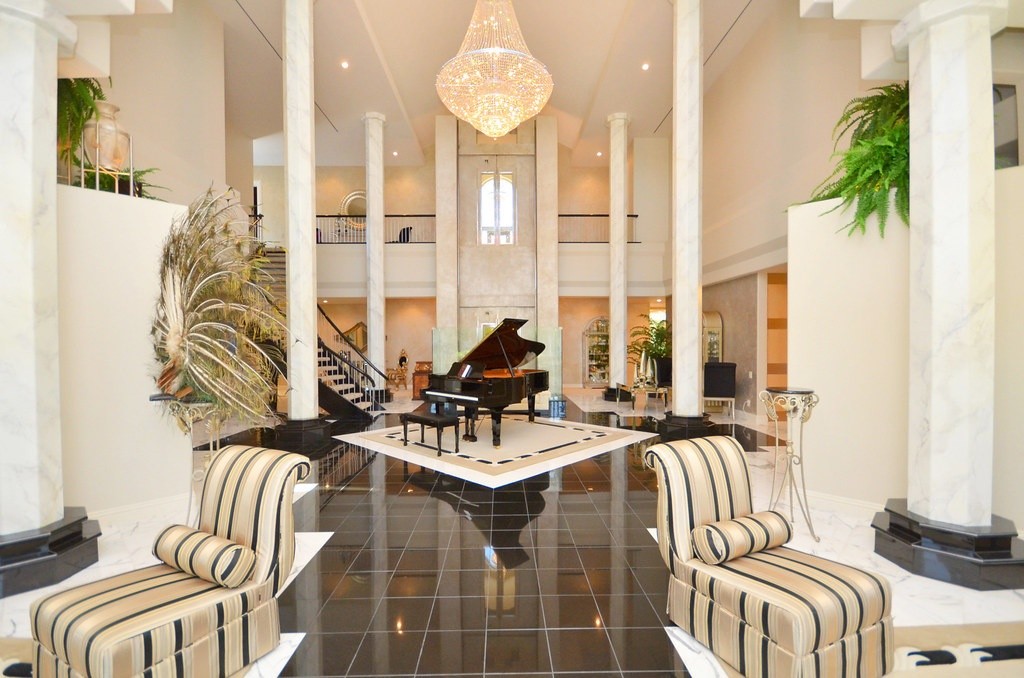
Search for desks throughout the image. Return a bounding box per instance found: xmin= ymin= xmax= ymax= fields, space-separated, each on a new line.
xmin=189 ymin=400 xmax=226 ymax=520
xmin=760 ymin=387 xmax=821 ymax=543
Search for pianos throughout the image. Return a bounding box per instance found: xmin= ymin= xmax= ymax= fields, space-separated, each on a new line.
xmin=419 ymin=317 xmax=550 ymax=448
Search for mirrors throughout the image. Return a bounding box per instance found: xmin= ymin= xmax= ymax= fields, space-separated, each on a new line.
xmin=333 ymin=322 xmax=387 ymax=353
xmin=340 ymin=191 xmax=367 ymax=230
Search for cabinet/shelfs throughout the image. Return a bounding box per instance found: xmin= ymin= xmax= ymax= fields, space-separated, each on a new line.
xmin=582 ymin=316 xmax=609 ymax=389
xmin=702 ymin=311 xmax=723 ymax=411
xmin=411 ymin=362 xmax=433 ymax=401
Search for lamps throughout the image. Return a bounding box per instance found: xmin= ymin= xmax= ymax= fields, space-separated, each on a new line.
xmin=435 ymin=0 xmax=554 ymax=138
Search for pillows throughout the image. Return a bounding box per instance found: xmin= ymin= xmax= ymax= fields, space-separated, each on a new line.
xmin=690 ymin=510 xmax=794 ymax=565
xmin=151 ymin=523 xmax=256 ymax=589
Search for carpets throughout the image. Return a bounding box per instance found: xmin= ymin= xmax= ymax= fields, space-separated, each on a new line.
xmin=330 ymin=412 xmax=660 ymax=488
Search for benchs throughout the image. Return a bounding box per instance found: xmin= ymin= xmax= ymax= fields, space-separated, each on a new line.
xmin=400 ymin=412 xmax=460 ymax=456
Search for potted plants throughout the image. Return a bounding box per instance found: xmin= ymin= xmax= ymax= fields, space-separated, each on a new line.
xmin=628 ymin=314 xmax=672 ymax=398
xmin=57 ymin=76 xmax=172 ymax=203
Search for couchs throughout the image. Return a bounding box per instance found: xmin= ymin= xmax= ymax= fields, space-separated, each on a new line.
xmin=30 ymin=445 xmax=313 ymax=678
xmin=644 ymin=435 xmax=895 ymax=678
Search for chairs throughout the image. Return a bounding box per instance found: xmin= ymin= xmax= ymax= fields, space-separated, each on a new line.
xmin=705 ymin=361 xmax=737 ymax=420
xmin=652 ymin=356 xmax=672 ymax=400
xmin=386 ymin=349 xmax=409 ymax=390
xmin=398 ymin=226 xmax=412 ymax=242
